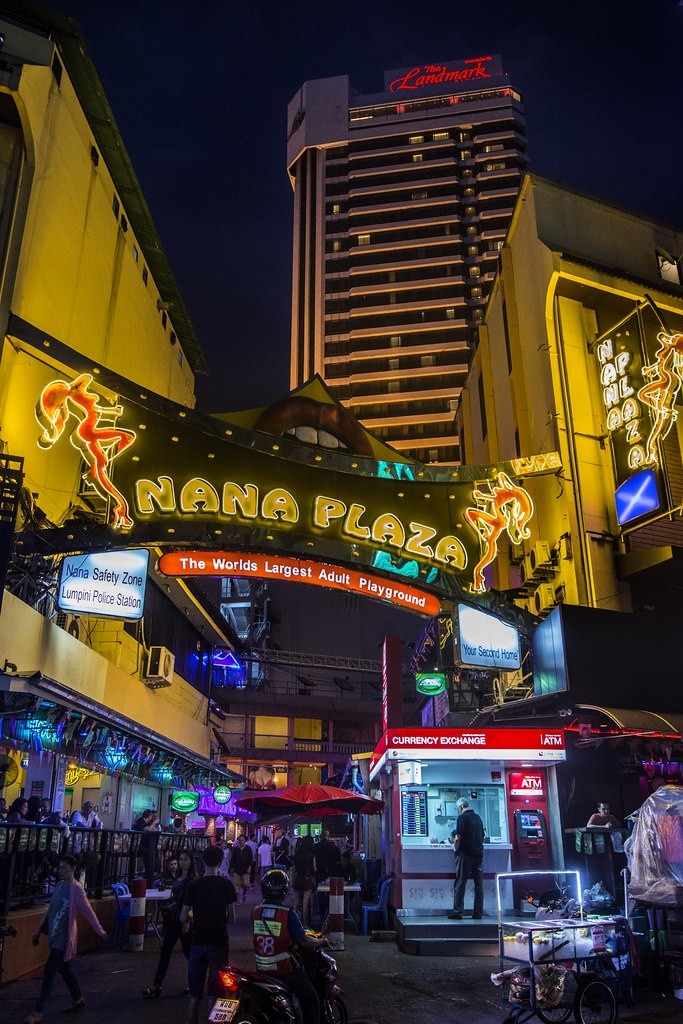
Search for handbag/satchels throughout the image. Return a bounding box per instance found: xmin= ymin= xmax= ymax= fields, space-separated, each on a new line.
xmin=508 ymin=964 xmax=530 ymax=1005
xmin=529 ymin=964 xmax=568 ymax=1009
xmin=287 ymin=948 xmax=305 ymax=974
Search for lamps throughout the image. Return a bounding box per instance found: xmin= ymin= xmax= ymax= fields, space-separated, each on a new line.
xmin=398 ymin=760 xmax=421 ymax=789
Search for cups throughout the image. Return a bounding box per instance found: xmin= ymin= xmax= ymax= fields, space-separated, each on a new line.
xmin=97 ymin=821 xmax=104 ymax=829
xmin=117 ymin=820 xmax=124 ymax=830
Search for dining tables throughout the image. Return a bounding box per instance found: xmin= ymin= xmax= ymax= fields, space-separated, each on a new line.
xmin=118 ymin=888 xmax=174 ymax=947
xmin=318 ymin=882 xmax=361 ymax=933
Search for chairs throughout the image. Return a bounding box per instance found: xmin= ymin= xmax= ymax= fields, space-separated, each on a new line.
xmin=358 ymin=877 xmax=392 ymax=936
xmin=155 ymin=878 xmax=162 ymax=929
xmin=111 ymin=883 xmax=130 ymax=944
xmin=117 ymin=883 xmax=131 ymax=941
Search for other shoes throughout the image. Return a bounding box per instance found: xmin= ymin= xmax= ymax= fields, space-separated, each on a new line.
xmin=472 ymin=915 xmax=481 ymax=919
xmin=243 ymin=896 xmax=247 ymax=903
xmin=24 ymin=1012 xmax=46 ymax=1023
xmin=61 ymin=998 xmax=87 ymax=1012
xmin=448 ymin=912 xmax=462 ymax=919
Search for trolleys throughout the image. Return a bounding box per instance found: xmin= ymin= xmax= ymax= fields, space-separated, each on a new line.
xmin=497 ymin=915 xmax=639 ymax=1024
xmin=572 ymin=829 xmax=634 ymax=891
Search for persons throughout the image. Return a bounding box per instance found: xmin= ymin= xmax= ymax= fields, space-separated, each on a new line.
xmin=585 ymin=800 xmax=621 ymax=896
xmin=180 ymin=846 xmax=239 ymax=1023
xmin=448 ymin=797 xmax=485 ymax=919
xmin=21 ymin=854 xmax=108 ymax=1023
xmin=250 ymin=870 xmax=332 ymax=1024
xmin=440 ymin=830 xmax=456 ymax=844
xmin=142 ymin=849 xmax=195 ymax=998
xmin=0 ymin=797 xmax=356 ymax=931
xmin=245 ymin=763 xmax=277 ymax=791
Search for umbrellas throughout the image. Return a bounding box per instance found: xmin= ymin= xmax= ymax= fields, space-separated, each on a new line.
xmin=232 ymin=782 xmax=387 ymax=816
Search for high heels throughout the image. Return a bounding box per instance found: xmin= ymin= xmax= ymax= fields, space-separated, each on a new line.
xmin=185 ymin=987 xmax=190 ymax=993
xmin=142 ymin=986 xmax=163 ymax=997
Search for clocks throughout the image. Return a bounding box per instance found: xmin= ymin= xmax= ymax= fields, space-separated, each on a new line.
xmin=651 ymin=775 xmax=666 ymax=794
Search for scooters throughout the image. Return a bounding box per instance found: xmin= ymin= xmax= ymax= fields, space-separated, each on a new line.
xmin=209 ymin=912 xmax=347 ymax=1024
xmin=524 ymin=875 xmax=619 ymax=920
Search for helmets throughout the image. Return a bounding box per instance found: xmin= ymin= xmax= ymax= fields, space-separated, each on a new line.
xmin=260 ymin=869 xmax=290 ymax=899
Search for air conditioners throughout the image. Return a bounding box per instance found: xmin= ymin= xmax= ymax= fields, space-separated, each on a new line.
xmin=520 ymin=539 xmax=556 ymax=616
xmin=146 ymin=646 xmax=175 ymax=686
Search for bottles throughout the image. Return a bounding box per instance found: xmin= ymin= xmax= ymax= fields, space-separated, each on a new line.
xmin=91 ymin=819 xmax=97 ymax=828
xmin=64 ymin=809 xmax=70 ymax=818
xmin=157 ymin=878 xmax=166 ymax=892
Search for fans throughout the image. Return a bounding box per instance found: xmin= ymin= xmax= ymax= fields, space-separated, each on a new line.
xmin=0 ymin=754 xmax=18 ymax=790
